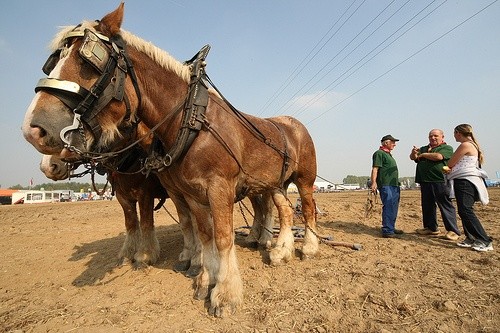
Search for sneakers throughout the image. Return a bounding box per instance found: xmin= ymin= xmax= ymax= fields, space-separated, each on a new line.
xmin=457 ymin=242 xmax=472 ymax=247
xmin=382 ymin=234 xmax=398 ymax=238
xmin=471 ymin=242 xmax=494 ymax=251
xmin=416 ymin=229 xmax=441 ymax=234
xmin=444 ymin=231 xmax=458 ymax=240
xmin=394 ymin=230 xmax=403 ymax=234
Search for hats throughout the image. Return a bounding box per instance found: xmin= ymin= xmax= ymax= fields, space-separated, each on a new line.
xmin=381 ymin=135 xmax=399 ymax=142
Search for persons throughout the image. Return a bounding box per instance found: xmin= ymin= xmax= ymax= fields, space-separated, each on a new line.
xmin=409 ymin=129 xmax=461 ymax=240
xmin=447 ymin=124 xmax=494 ymax=251
xmin=370 ymin=135 xmax=403 ymax=238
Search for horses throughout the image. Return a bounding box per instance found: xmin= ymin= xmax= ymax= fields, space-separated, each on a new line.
xmin=23 ymin=1 xmax=316 ymax=321
